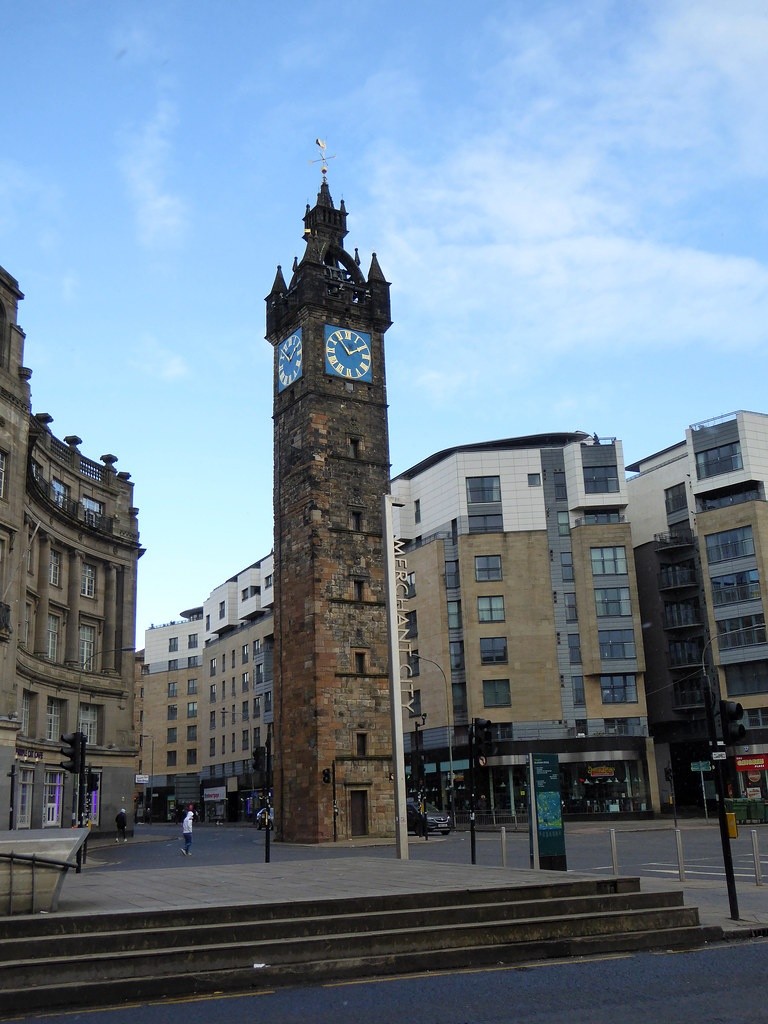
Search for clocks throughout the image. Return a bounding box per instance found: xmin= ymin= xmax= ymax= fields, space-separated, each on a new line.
xmin=279 ymin=334 xmax=302 ymax=386
xmin=324 ymin=329 xmax=373 ymax=382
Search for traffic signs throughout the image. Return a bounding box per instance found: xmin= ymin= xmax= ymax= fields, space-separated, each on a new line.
xmin=690 ymin=761 xmax=712 ymax=772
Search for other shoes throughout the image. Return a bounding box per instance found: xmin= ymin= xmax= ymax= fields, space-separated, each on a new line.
xmin=124 ymin=839 xmax=128 ymax=843
xmin=181 ymin=848 xmax=187 ymax=856
xmin=116 ymin=838 xmax=119 ymax=843
xmin=188 ymin=852 xmax=192 ymax=856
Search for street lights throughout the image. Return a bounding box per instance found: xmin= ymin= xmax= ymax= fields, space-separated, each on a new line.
xmin=411 ymin=653 xmax=457 ymax=829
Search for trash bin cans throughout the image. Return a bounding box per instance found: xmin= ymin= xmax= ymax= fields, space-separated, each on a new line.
xmin=723 ymin=798 xmax=768 ymax=824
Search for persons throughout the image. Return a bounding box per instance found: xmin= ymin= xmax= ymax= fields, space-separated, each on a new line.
xmin=147 ymin=808 xmax=152 ymax=824
xmin=180 ymin=812 xmax=194 ymax=856
xmin=115 ymin=809 xmax=127 ymax=842
xmin=168 ymin=809 xmax=202 ymax=825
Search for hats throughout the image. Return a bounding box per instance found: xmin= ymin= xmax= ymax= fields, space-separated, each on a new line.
xmin=121 ymin=809 xmax=126 ymax=813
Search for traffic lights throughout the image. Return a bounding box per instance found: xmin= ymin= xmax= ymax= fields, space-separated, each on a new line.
xmin=252 ymin=747 xmax=264 ymax=772
xmin=90 ymin=774 xmax=99 ymax=792
xmin=474 ymin=717 xmax=493 ymax=755
xmin=322 ymin=768 xmax=331 ymax=784
xmin=60 ymin=732 xmax=81 ymax=774
xmin=665 ymin=767 xmax=673 ymax=782
xmin=721 ymin=701 xmax=748 ymax=745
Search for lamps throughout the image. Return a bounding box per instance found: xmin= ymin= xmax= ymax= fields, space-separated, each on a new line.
xmin=56 ymin=684 xmax=63 ymax=693
xmin=31 ymin=674 xmax=36 ymax=684
xmin=8 ymin=711 xmax=18 ymax=720
xmin=90 ymin=690 xmax=96 ymax=701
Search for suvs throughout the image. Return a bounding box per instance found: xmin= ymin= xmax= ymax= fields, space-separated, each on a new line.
xmin=406 ymin=802 xmax=453 ymax=836
